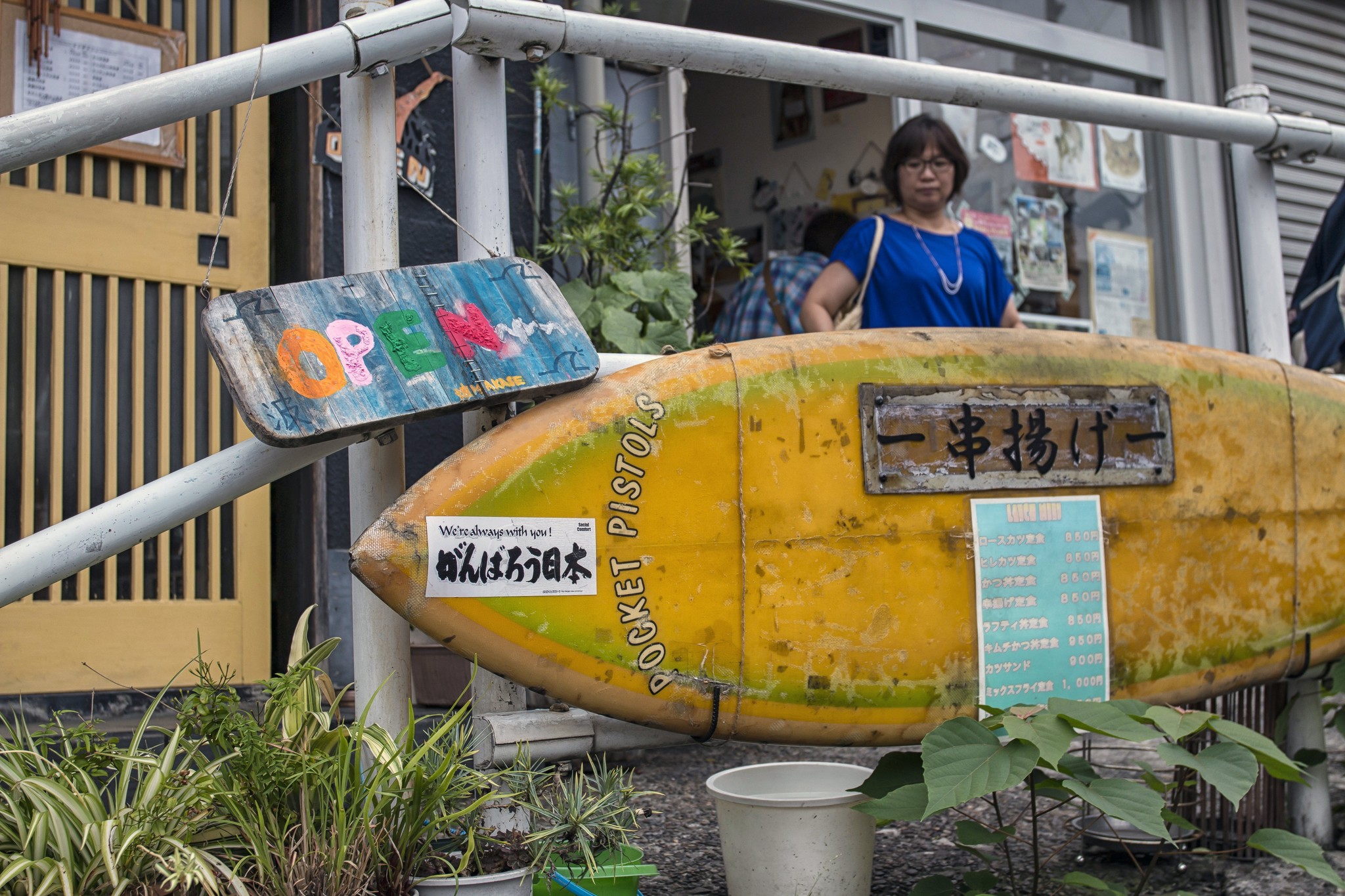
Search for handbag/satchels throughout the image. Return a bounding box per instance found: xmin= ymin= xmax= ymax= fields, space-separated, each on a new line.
xmin=832 ymin=213 xmax=883 ymax=332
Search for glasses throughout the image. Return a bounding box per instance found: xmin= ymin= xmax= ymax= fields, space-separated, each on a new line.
xmin=903 ymin=155 xmax=953 ymax=176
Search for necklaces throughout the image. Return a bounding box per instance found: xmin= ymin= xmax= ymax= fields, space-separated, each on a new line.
xmin=907 ymin=213 xmax=963 ymax=294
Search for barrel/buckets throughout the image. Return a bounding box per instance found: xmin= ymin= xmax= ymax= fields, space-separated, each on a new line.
xmin=410 ymin=644 xmax=471 ymax=707
xmin=533 ymin=840 xmax=660 ymax=896
xmin=706 ymin=761 xmax=875 ymax=896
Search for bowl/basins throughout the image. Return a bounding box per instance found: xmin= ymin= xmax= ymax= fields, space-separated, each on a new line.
xmin=1072 ymin=814 xmax=1195 ymax=853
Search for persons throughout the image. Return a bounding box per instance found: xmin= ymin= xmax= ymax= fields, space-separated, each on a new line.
xmin=799 ymin=112 xmax=1028 ymax=333
xmin=714 ymin=207 xmax=859 ymax=345
xmin=1015 ymin=196 xmax=1064 ymax=263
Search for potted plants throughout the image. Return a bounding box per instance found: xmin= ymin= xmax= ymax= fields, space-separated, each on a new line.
xmin=398 ymin=739 xmax=664 ymax=896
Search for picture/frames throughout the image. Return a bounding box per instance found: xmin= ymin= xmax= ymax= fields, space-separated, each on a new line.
xmin=818 ymin=27 xmax=867 ymax=112
xmin=768 ymin=81 xmax=814 ymax=149
